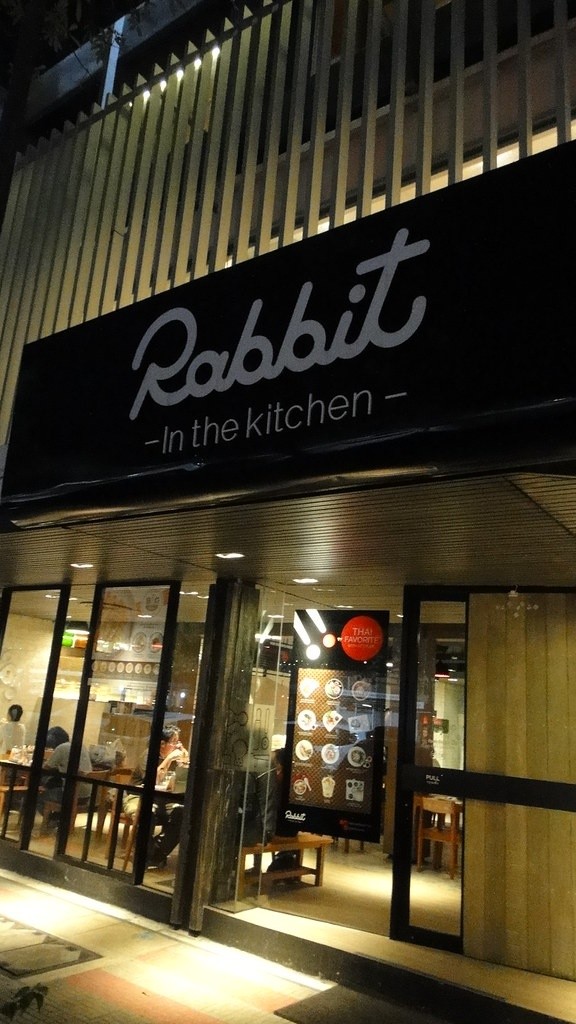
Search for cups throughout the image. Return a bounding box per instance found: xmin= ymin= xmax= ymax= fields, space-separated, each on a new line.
xmin=322 ymin=779 xmax=335 ymax=798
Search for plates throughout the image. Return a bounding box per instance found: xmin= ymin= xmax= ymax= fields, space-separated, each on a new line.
xmin=296 ymin=679 xmax=370 ymax=767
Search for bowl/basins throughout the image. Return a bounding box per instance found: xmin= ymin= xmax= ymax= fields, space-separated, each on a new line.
xmin=293 ymin=780 xmax=307 ymax=795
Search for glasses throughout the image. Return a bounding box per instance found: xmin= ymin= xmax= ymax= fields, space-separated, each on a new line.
xmin=169 ymin=740 xmax=182 ymax=745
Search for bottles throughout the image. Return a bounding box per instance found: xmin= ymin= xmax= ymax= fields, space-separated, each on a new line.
xmin=120 ymin=687 xmax=128 ymax=703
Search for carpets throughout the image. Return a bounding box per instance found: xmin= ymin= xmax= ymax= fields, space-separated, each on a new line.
xmin=272 ymin=982 xmax=447 ymax=1024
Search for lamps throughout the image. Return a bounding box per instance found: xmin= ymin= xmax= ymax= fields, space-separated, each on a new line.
xmin=65 ymin=621 xmax=90 ymax=635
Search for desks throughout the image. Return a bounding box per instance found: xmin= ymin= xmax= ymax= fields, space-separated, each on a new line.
xmin=155 ymin=767 xmax=188 ymax=795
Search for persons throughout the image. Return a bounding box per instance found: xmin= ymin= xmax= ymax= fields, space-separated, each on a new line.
xmin=37 ymin=727 xmax=93 ymax=827
xmin=147 ymin=728 xmax=276 ymax=866
xmin=0 ymin=704 xmax=26 ymax=755
xmin=122 ymin=724 xmax=188 ymax=866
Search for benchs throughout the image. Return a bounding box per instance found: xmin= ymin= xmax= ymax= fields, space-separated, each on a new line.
xmin=238 ymin=834 xmax=333 ymax=901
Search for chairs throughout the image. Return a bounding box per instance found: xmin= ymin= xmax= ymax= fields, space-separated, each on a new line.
xmin=43 ymin=769 xmax=144 ymax=870
xmin=0 ymin=754 xmax=34 ymax=826
xmin=417 ymin=797 xmax=463 ymax=880
